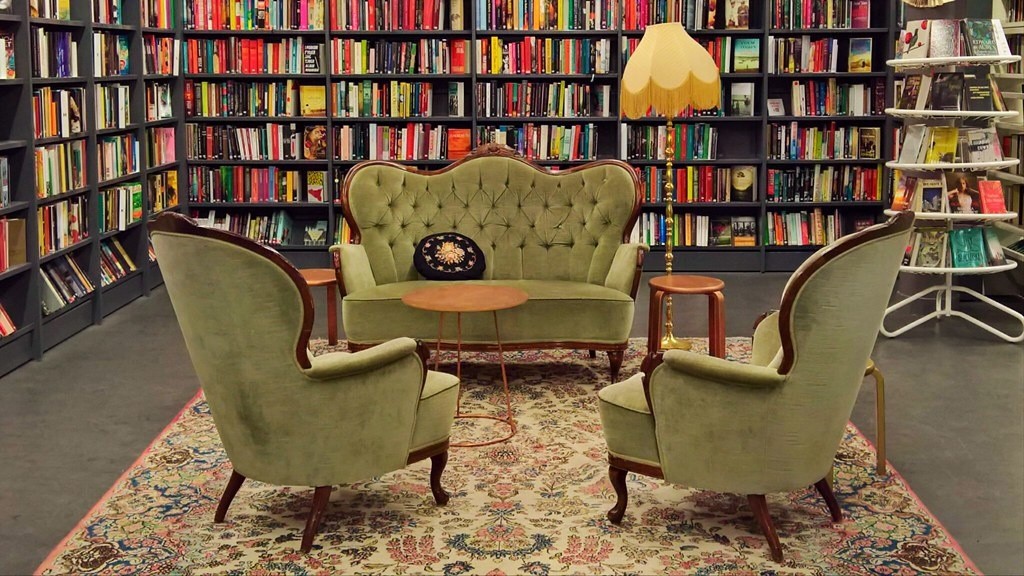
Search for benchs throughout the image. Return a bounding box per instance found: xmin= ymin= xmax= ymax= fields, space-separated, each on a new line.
xmin=329 ymin=144 xmax=650 ymax=386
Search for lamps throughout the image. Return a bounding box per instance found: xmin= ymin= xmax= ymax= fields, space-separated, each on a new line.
xmin=620 ymin=22 xmax=721 ymax=351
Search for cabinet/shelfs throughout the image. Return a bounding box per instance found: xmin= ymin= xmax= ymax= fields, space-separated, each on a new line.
xmin=881 ymin=55 xmax=1024 ymax=343
xmin=0 ymin=0 xmax=1024 ymax=378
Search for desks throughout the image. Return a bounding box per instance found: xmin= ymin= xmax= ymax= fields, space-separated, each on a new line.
xmin=400 ymin=284 xmax=528 ymax=447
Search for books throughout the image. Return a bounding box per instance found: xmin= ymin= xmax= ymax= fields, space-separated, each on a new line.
xmin=991 ymin=0 xmax=1024 ymax=259
xmin=328 ymin=0 xmax=889 ymax=253
xmin=892 ymin=0 xmax=1015 ymax=270
xmin=0 ymin=0 xmax=329 ymax=341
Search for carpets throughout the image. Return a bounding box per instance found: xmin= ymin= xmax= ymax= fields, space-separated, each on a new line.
xmin=31 ymin=337 xmax=983 ymax=576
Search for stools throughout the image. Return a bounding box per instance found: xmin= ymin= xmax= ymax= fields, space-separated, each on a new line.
xmin=299 ymin=268 xmax=338 ymax=345
xmin=647 ymin=275 xmax=726 ymax=371
xmin=827 ymin=357 xmax=887 ymax=493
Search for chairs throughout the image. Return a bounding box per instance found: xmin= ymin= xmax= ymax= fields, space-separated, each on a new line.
xmin=598 ymin=209 xmax=913 ymax=563
xmin=146 ymin=212 xmax=461 ymax=553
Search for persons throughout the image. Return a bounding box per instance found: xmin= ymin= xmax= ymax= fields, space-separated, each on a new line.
xmin=947 ymin=177 xmax=979 ymax=215
